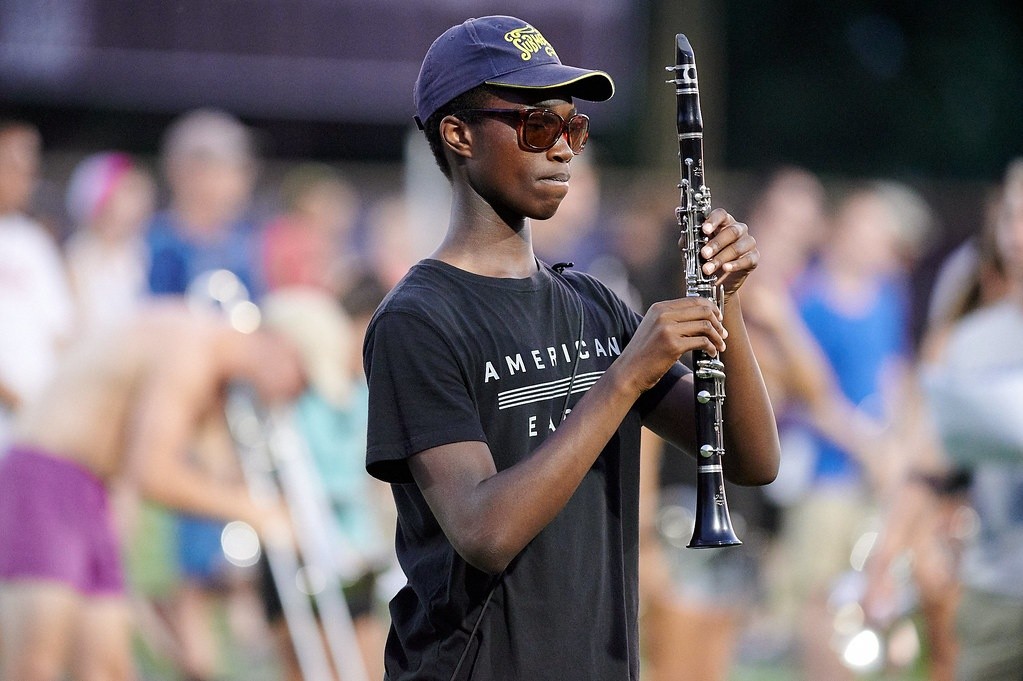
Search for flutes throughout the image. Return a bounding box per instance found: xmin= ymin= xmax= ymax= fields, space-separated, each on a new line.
xmin=667 ymin=29 xmax=745 ymax=551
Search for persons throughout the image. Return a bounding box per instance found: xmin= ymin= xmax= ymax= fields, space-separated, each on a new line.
xmin=531 ymin=143 xmax=1023 ymax=681
xmin=362 ymin=13 xmax=780 ymax=681
xmin=0 ymin=108 xmax=449 ymax=681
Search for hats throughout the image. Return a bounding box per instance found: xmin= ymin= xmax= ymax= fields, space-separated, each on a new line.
xmin=413 ymin=15 xmax=615 ymax=130
xmin=65 ymin=152 xmax=132 ymax=229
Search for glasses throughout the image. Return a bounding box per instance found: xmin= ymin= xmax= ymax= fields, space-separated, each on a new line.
xmin=452 ymin=106 xmax=590 ymax=155
